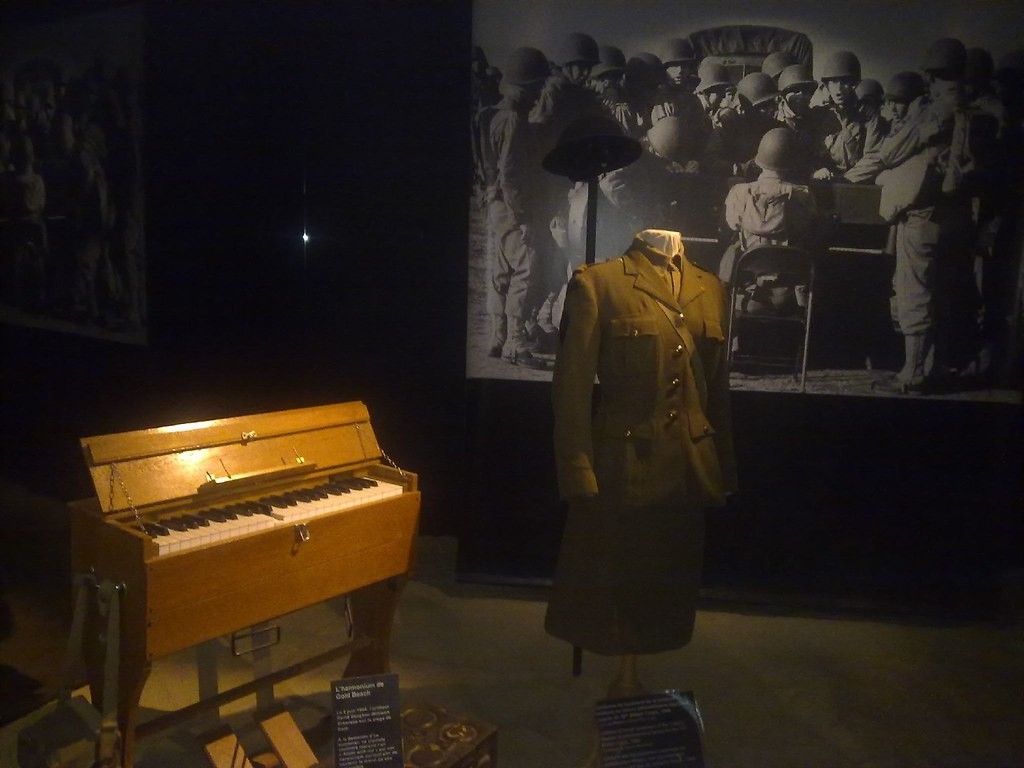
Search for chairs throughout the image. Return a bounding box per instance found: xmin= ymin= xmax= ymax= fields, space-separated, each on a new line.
xmin=727 ymin=244 xmax=817 ymax=393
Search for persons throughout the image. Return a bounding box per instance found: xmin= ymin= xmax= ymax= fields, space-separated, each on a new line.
xmin=1 ymin=58 xmax=142 ymax=333
xmin=468 ymin=32 xmax=1024 ymax=393
xmin=542 ymin=230 xmax=738 ymax=739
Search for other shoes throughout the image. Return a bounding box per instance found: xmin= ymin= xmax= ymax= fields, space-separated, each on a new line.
xmin=501 ymin=347 xmax=547 ymax=370
xmin=489 ymin=347 xmax=502 ymax=360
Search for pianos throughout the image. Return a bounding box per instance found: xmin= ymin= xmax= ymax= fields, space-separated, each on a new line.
xmin=675 ymin=172 xmax=887 ymax=258
xmin=64 ymin=396 xmax=424 ymax=768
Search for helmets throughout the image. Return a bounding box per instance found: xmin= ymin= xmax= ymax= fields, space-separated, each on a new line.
xmin=472 ymin=28 xmax=1024 ymax=200
xmin=541 ymin=116 xmax=644 ymax=181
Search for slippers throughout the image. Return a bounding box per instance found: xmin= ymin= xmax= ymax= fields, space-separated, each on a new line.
xmin=871 ymin=373 xmax=929 ymax=396
